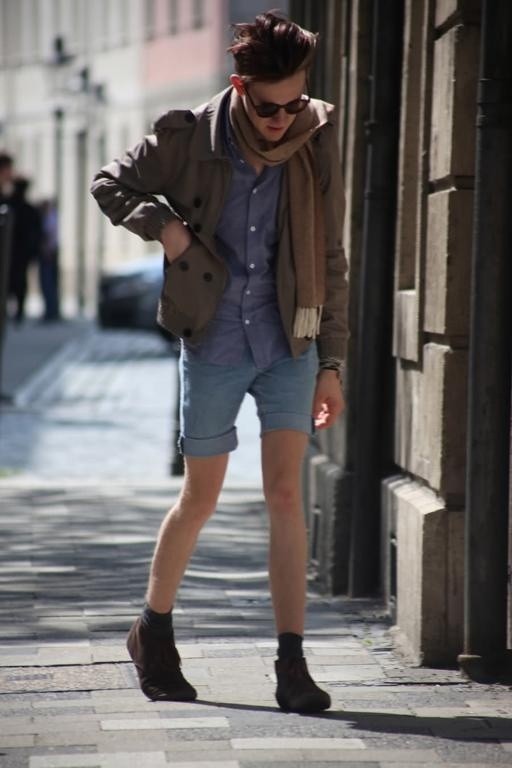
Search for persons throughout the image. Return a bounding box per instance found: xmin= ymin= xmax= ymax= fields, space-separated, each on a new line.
xmin=0 ymin=153 xmax=58 ymax=320
xmin=89 ymin=12 xmax=351 ymax=712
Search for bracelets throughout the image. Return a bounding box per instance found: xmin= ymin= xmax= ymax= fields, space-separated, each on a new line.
xmin=321 ymin=362 xmax=338 ymax=371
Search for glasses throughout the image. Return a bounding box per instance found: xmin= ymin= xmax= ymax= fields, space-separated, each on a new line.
xmin=242 ymin=79 xmax=312 ymax=119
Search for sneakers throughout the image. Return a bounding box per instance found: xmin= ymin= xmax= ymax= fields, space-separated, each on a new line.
xmin=272 ymin=655 xmax=332 ymax=714
xmin=125 ymin=615 xmax=198 ymax=702
